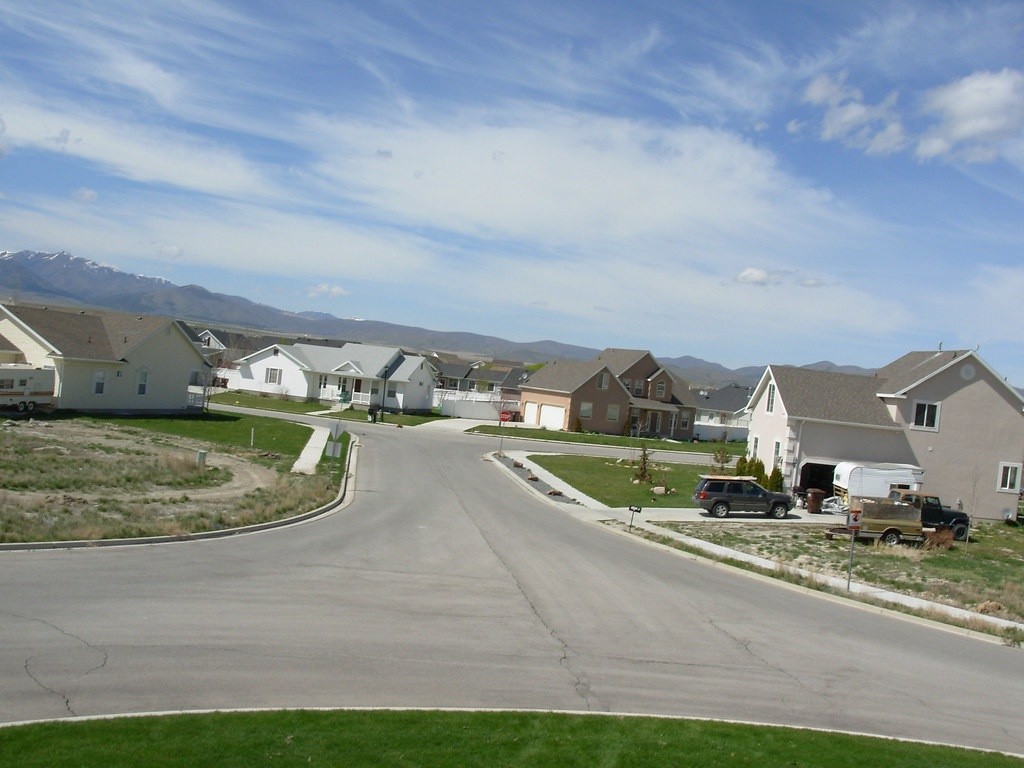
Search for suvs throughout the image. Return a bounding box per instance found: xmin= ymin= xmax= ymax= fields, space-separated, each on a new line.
xmin=887 ymin=488 xmax=971 ymax=539
xmin=691 ymin=479 xmax=795 ymax=519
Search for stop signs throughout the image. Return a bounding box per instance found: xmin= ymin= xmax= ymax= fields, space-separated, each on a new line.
xmin=499 ymin=411 xmax=512 ymax=422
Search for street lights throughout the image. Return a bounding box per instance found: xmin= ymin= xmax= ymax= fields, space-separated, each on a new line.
xmin=378 ymin=365 xmax=390 ymax=421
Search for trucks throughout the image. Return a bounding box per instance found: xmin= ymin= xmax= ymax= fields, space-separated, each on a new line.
xmin=0 ymin=363 xmax=56 ymax=412
